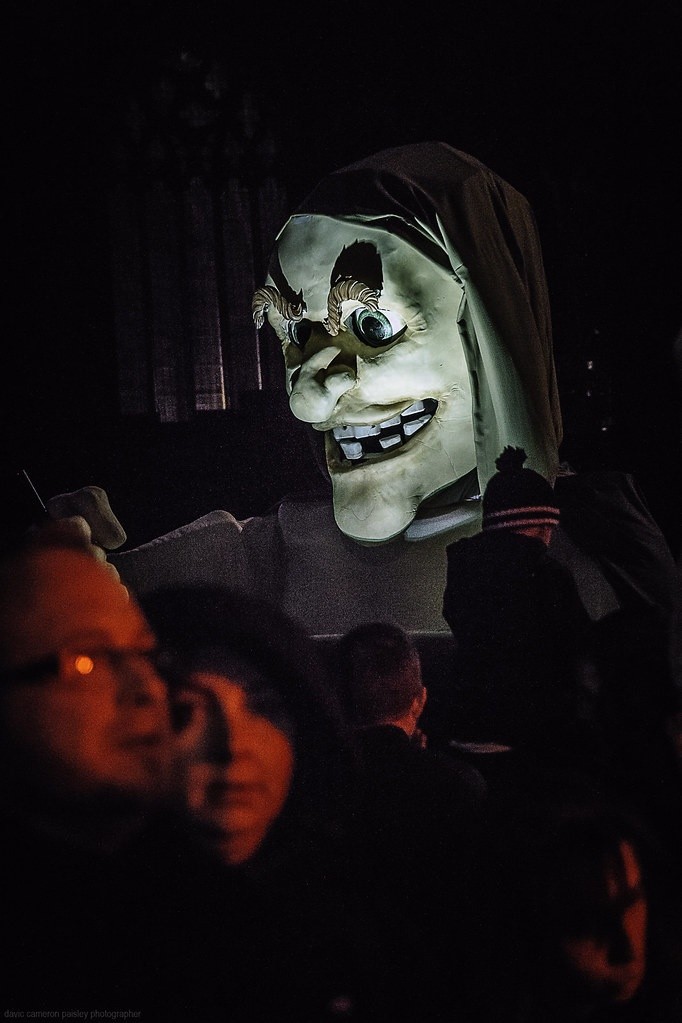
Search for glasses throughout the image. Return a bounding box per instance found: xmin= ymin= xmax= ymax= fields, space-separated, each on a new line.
xmin=0 ymin=639 xmax=179 ymax=691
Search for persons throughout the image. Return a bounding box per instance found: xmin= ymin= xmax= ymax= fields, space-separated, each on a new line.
xmin=0 ymin=446 xmax=682 ymax=1023
xmin=48 ymin=138 xmax=682 ymax=742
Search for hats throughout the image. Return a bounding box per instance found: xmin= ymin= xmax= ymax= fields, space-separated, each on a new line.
xmin=482 ymin=444 xmax=562 ymax=533
xmin=142 ymin=586 xmax=352 ymax=772
